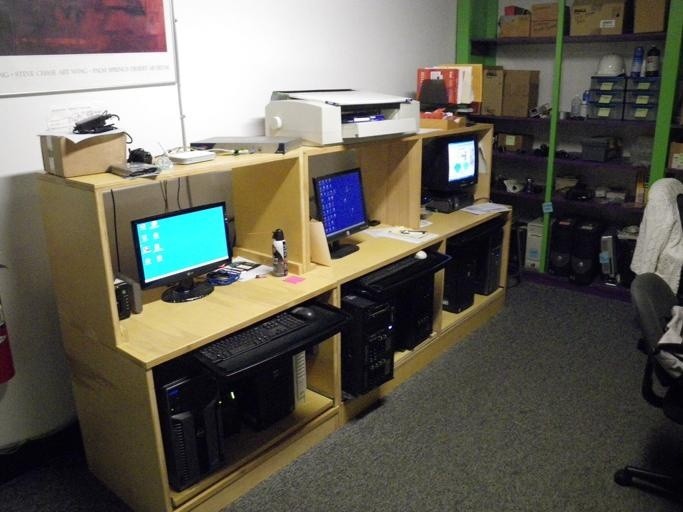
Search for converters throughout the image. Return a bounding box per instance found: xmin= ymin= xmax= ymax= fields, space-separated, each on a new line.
xmin=75 ymin=114 xmax=106 ymax=130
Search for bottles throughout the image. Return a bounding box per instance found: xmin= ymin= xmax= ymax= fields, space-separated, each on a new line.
xmin=571 ymin=87 xmax=589 ymax=118
xmin=645 ymin=45 xmax=660 ymax=77
xmin=631 ymin=44 xmax=644 ymax=78
xmin=271 ymin=229 xmax=288 ymax=278
xmin=634 ymin=166 xmax=649 ymax=205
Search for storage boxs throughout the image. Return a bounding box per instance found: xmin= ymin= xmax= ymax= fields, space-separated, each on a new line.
xmin=482 ymin=1 xmax=668 ymax=117
xmin=668 ymin=143 xmax=683 ymax=170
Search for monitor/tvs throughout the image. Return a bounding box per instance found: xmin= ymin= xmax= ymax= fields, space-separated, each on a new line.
xmin=422 ymin=136 xmax=478 ymax=193
xmin=130 ymin=202 xmax=231 ymax=303
xmin=312 ymin=167 xmax=368 ymax=258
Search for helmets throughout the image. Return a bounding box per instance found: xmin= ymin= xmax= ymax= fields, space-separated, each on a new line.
xmin=596 ymin=52 xmax=625 ymax=76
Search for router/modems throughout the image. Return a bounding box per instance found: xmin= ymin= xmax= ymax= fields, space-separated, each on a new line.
xmin=158 ymin=135 xmax=216 ymax=163
xmin=115 ymin=272 xmax=142 ymax=313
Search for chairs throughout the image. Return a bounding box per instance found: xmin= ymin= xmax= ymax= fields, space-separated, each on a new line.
xmin=630 ymin=178 xmax=683 ymax=296
xmin=615 ymin=273 xmax=683 ymax=500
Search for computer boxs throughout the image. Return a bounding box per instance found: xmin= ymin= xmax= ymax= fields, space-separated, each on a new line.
xmin=469 ymin=228 xmax=502 ymax=296
xmin=152 ymin=369 xmax=225 ymax=492
xmin=341 ymin=295 xmax=393 ymax=396
xmin=523 ymin=221 xmax=543 ymax=272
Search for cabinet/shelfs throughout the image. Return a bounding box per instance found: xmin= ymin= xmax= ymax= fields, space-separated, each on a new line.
xmin=456 ymin=1 xmax=682 ymax=303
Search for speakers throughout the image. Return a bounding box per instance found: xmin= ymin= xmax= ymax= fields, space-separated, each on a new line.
xmin=550 ymin=218 xmax=573 ymax=275
xmin=570 ymin=224 xmax=600 ymax=278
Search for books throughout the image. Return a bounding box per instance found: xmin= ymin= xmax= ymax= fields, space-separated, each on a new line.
xmin=415 ymin=62 xmax=484 ymax=106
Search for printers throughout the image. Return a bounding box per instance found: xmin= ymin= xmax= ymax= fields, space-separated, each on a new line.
xmin=265 ymin=88 xmax=420 ymax=146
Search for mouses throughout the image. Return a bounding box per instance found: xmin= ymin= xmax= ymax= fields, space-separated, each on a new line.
xmin=415 ymin=251 xmax=427 ymax=260
xmin=292 ymin=306 xmax=317 ymax=323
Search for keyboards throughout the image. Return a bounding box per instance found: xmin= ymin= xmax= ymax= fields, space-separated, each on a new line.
xmin=358 ymin=255 xmax=417 ymax=287
xmin=199 ymin=313 xmax=307 ymax=364
